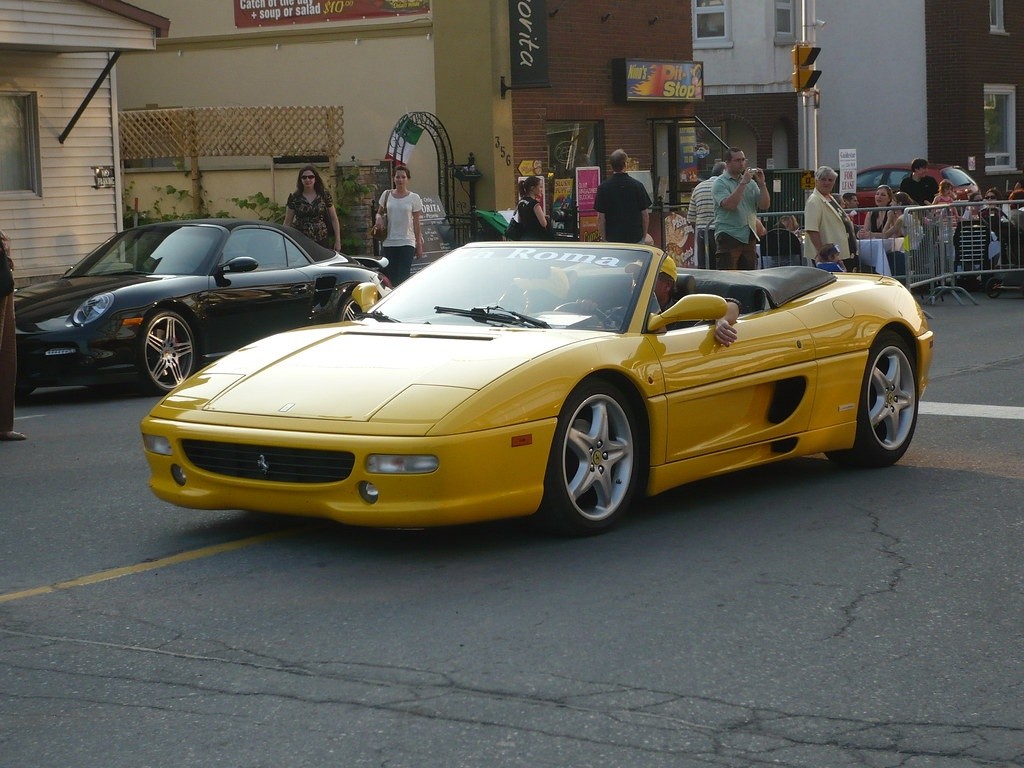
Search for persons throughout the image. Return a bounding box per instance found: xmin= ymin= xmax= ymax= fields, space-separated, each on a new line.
xmin=518 ymin=176 xmax=550 ymax=240
xmin=283 ymin=166 xmax=341 ymax=253
xmin=593 ymin=149 xmax=653 ymax=245
xmin=0 ymin=229 xmax=26 ymax=440
xmin=375 ymin=166 xmax=422 ymax=287
xmin=575 ymin=256 xmax=740 ymax=348
xmin=804 ymin=158 xmax=1024 ymax=282
xmin=768 ymin=215 xmax=798 ymax=267
xmin=686 ymin=147 xmax=770 ymax=270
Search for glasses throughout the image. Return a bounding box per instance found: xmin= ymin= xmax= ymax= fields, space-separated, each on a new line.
xmin=300 ymin=175 xmax=315 ymax=180
xmin=983 ymin=195 xmax=998 ymax=200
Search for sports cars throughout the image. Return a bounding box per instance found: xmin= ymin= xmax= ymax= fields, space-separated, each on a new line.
xmin=10 ymin=218 xmax=397 ymax=399
xmin=139 ymin=237 xmax=935 ymax=530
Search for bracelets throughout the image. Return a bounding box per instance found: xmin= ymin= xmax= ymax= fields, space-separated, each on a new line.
xmin=758 ymin=183 xmax=766 ymax=187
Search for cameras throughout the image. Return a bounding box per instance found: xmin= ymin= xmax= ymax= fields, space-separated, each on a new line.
xmin=748 ymin=168 xmax=758 ymax=173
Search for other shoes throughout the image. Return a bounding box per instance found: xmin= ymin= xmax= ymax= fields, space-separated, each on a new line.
xmin=0 ymin=430 xmax=27 ymax=441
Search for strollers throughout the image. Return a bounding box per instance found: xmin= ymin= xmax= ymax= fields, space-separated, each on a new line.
xmin=979 ymin=206 xmax=1024 ymax=299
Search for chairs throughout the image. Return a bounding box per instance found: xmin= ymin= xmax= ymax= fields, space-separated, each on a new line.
xmin=624 ymin=271 xmax=698 ymax=300
xmin=953 ymin=220 xmax=991 ymax=293
xmin=760 ymin=229 xmax=803 ymax=270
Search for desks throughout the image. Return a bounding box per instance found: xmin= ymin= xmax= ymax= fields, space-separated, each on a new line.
xmin=755 ymin=237 xmax=906 ymax=276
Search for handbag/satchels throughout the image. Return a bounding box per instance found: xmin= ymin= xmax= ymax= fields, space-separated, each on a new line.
xmin=370 ymin=190 xmax=388 ymax=242
xmin=504 ymin=204 xmax=522 ymax=242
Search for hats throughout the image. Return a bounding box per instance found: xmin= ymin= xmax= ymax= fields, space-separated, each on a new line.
xmin=513 ymin=267 xmax=569 ymax=300
xmin=625 ymin=252 xmax=679 ymax=283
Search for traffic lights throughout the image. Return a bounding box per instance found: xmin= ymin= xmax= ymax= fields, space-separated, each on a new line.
xmin=791 ymin=46 xmax=822 ymax=93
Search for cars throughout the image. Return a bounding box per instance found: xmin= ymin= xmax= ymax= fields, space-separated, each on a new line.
xmin=850 ymin=161 xmax=982 ymax=231
